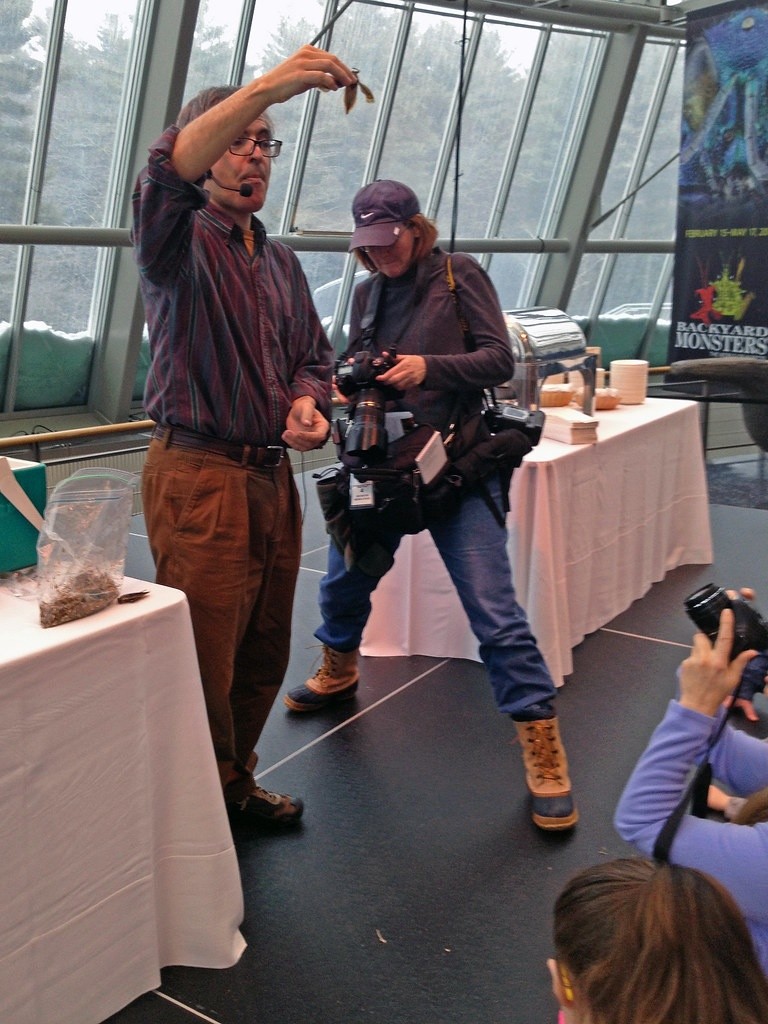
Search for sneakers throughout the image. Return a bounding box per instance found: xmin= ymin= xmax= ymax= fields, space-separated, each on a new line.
xmin=226 ymin=787 xmax=304 ymax=829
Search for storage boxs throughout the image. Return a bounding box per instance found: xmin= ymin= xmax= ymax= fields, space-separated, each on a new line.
xmin=0 ymin=457 xmax=46 ymax=574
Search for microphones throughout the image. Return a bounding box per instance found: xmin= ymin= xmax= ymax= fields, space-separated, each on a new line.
xmin=209 ymin=176 xmax=253 ymax=197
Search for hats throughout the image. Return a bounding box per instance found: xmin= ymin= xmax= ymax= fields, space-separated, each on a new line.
xmin=348 ymin=178 xmax=420 ymax=254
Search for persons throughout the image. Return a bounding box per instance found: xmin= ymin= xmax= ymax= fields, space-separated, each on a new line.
xmin=547 ymin=859 xmax=768 ymax=1024
xmin=132 ymin=44 xmax=358 ymax=849
xmin=284 ymin=180 xmax=579 ymax=831
xmin=614 ymin=588 xmax=768 ymax=982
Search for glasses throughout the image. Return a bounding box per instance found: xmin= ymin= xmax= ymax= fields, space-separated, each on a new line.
xmin=228 ymin=137 xmax=283 ymax=158
xmin=360 ymin=224 xmax=412 ymax=253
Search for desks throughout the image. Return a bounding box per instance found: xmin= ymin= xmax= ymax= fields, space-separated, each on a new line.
xmin=0 ymin=565 xmax=247 ymax=1024
xmin=357 ymin=397 xmax=714 ymax=688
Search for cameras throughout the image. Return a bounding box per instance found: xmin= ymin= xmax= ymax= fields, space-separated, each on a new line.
xmin=682 ymin=582 xmax=768 ymax=667
xmin=480 ymin=404 xmax=546 ymax=447
xmin=333 ymin=352 xmax=403 ymax=455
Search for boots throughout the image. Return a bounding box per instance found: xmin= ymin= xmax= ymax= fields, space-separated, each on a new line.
xmin=513 ymin=704 xmax=580 ymax=830
xmin=284 ymin=643 xmax=361 ymax=711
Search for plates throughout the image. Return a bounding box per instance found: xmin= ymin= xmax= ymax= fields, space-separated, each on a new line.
xmin=536 ymin=367 xmax=606 ymax=389
xmin=610 ymin=360 xmax=649 ymax=404
